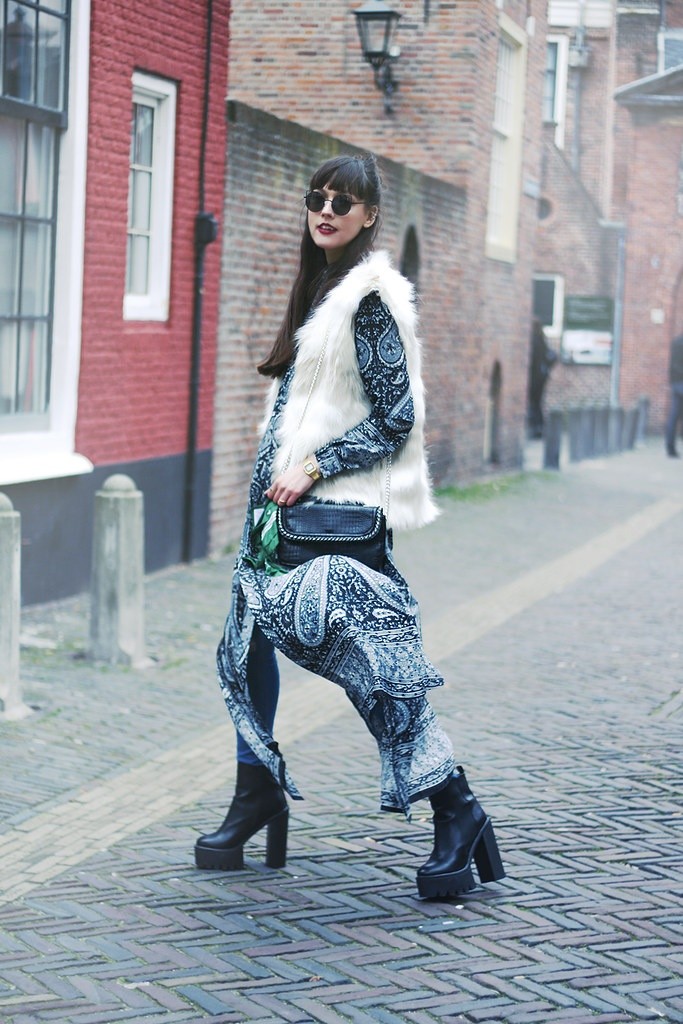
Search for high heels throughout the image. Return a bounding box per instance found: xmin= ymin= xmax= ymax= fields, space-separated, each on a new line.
xmin=415 ymin=766 xmax=506 ymax=897
xmin=192 ymin=762 xmax=290 ymax=871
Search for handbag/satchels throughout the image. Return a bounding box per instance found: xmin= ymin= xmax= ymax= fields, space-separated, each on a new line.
xmin=270 ymin=500 xmax=386 ymax=573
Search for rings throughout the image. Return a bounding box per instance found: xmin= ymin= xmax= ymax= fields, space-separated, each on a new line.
xmin=270 ymin=488 xmax=275 ymax=493
xmin=279 ymin=498 xmax=286 ymax=503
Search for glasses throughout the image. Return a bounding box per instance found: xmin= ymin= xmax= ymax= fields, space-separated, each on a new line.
xmin=303 ymin=191 xmax=369 ymax=216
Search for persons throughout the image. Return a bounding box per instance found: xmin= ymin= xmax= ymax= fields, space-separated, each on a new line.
xmin=196 ymin=155 xmax=505 ymax=897
xmin=526 ymin=318 xmax=556 ymax=440
xmin=664 ymin=334 xmax=683 ymax=457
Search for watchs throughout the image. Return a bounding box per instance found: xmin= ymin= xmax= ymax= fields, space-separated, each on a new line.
xmin=303 ymin=458 xmax=319 ymax=480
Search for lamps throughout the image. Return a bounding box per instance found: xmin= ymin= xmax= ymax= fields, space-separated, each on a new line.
xmin=351 ymin=0 xmax=403 ymax=115
